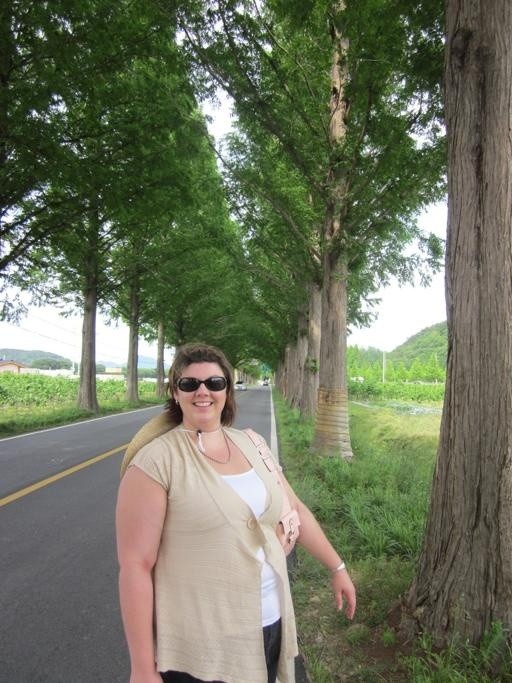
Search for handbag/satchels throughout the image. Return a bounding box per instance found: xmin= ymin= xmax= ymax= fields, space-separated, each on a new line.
xmin=269 ymin=469 xmax=300 ymax=558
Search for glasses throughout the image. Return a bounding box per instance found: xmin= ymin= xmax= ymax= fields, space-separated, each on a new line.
xmin=174 ymin=377 xmax=228 ymax=392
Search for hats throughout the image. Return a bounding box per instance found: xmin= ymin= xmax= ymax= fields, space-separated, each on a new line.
xmin=120 ymin=408 xmax=180 ymax=481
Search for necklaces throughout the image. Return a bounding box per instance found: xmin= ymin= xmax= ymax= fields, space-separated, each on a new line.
xmin=188 ymin=428 xmax=231 ymax=465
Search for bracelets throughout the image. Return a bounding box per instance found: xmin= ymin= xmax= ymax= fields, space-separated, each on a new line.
xmin=331 ymin=562 xmax=346 ymax=573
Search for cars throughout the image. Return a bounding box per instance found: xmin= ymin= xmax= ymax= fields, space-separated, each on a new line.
xmin=234 ymin=381 xmax=248 ymax=389
xmin=263 ymin=382 xmax=268 ymax=385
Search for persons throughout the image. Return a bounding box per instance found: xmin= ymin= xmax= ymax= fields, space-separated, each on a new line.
xmin=114 ymin=343 xmax=358 ymax=682
